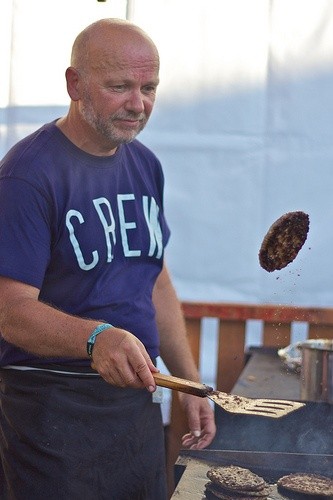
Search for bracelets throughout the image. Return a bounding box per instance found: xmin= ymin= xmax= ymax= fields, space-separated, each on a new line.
xmin=86 ymin=323 xmax=113 ymax=360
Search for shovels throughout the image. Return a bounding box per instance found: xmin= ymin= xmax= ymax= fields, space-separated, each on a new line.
xmin=91 ymin=361 xmax=306 ymax=419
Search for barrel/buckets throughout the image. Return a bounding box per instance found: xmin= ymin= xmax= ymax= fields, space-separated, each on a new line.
xmin=298 ymin=339 xmax=333 ymax=403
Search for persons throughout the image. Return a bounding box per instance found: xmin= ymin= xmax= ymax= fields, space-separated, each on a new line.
xmin=0 ymin=17 xmax=216 ymax=500
